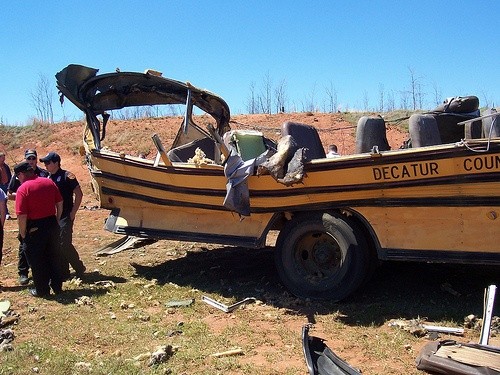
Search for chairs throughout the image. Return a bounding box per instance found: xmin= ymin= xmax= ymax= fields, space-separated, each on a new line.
xmin=481 ymin=109 xmax=500 ymax=138
xmin=355 ymin=115 xmax=392 ymax=153
xmin=281 ymin=121 xmax=327 ymax=159
xmin=225 ymin=129 xmax=266 ymax=168
xmin=406 ymin=114 xmax=442 ymax=148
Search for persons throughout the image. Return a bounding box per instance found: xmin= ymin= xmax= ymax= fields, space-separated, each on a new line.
xmin=0 ymin=167 xmax=8 ymax=266
xmin=44 ymin=152 xmax=86 ymax=281
xmin=6 ymin=148 xmax=50 ymax=285
xmin=0 ymin=152 xmax=12 ymax=220
xmin=14 ymin=161 xmax=63 ymax=300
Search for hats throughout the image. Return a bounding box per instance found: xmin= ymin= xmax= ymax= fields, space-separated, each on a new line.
xmin=13 ymin=162 xmax=30 ymax=174
xmin=39 ymin=152 xmax=61 ymax=163
xmin=25 ymin=148 xmax=37 ymax=159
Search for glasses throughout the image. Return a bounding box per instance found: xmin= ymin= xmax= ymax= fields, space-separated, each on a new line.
xmin=44 ymin=162 xmax=54 ymax=165
xmin=27 ymin=157 xmax=36 ymax=160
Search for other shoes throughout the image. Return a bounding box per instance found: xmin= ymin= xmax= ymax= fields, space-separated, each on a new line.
xmin=32 ymin=289 xmax=50 ymax=296
xmin=20 ymin=276 xmax=29 ymax=284
